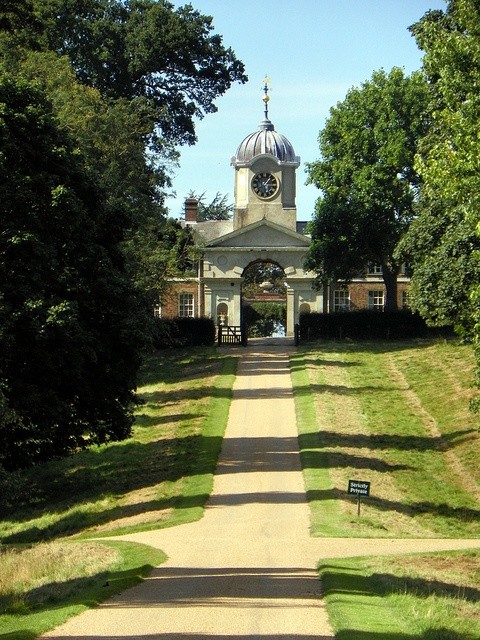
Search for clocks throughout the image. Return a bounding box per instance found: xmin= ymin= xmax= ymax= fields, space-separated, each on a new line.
xmin=250 ymin=169 xmax=280 ymax=201
